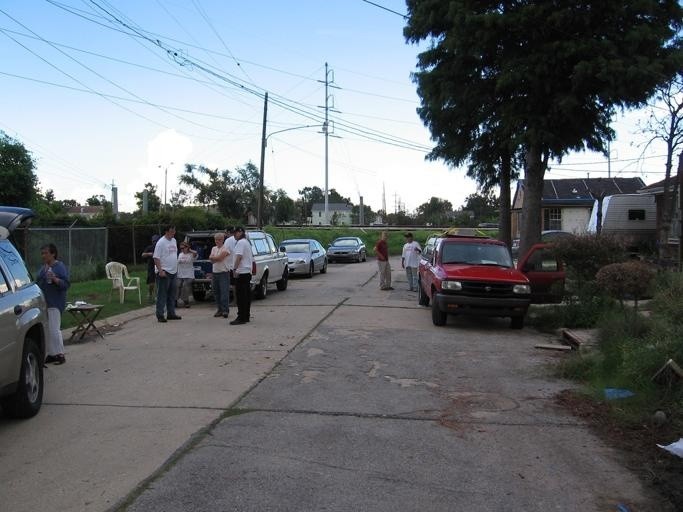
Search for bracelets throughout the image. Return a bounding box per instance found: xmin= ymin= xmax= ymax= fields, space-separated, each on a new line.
xmin=233 ymin=269 xmax=236 ymax=270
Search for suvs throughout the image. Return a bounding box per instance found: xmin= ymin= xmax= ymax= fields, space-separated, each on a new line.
xmin=0 ymin=205 xmax=47 ymax=420
xmin=184 ymin=228 xmax=289 ymax=302
xmin=417 ymin=236 xmax=567 ymax=329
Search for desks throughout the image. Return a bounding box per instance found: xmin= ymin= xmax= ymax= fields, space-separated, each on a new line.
xmin=65 ymin=303 xmax=105 ymax=345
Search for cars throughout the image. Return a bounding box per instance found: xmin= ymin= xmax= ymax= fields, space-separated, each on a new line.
xmin=278 ymin=238 xmax=328 ymax=278
xmin=512 ymin=229 xmax=581 ymax=255
xmin=326 ymin=236 xmax=366 ymax=263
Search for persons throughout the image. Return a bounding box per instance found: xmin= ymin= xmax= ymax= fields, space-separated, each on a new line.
xmin=209 ymin=232 xmax=231 ymax=318
xmin=152 ymin=223 xmax=181 ymax=322
xmin=141 ymin=234 xmax=161 ymax=303
xmin=174 ymin=241 xmax=199 ymax=308
xmin=35 ymin=243 xmax=71 ymax=365
xmin=401 ymin=233 xmax=421 ymax=292
xmin=230 ymin=227 xmax=253 ymax=325
xmin=373 ymin=231 xmax=394 ymax=290
xmin=222 ymin=225 xmax=238 ymax=307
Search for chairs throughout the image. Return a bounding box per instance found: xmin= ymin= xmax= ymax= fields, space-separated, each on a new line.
xmin=105 ymin=262 xmax=141 ymax=305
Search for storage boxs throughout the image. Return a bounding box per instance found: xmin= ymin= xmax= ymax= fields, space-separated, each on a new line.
xmin=192 ymin=258 xmax=212 ymax=279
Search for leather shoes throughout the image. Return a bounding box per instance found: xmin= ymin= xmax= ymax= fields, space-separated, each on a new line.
xmin=229 ymin=317 xmax=245 ymax=325
xmin=166 ymin=314 xmax=180 ymax=319
xmin=157 ymin=317 xmax=166 ymax=322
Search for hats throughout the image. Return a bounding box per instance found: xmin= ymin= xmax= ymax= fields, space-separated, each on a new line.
xmin=231 ymin=227 xmax=243 ymax=233
xmin=403 ymin=233 xmax=412 ymax=238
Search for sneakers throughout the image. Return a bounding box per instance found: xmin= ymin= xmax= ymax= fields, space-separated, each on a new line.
xmin=222 ymin=311 xmax=228 ymax=318
xmin=44 ymin=354 xmax=55 ymax=363
xmin=53 ymin=353 xmax=65 ymax=365
xmin=214 ymin=310 xmax=222 ymax=317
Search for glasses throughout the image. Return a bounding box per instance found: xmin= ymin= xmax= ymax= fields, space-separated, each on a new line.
xmin=179 ymin=247 xmax=185 ymax=250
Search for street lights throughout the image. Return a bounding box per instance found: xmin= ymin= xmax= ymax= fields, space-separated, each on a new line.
xmin=157 ymin=161 xmax=176 ymax=211
xmin=257 ymin=91 xmax=329 ymax=224
